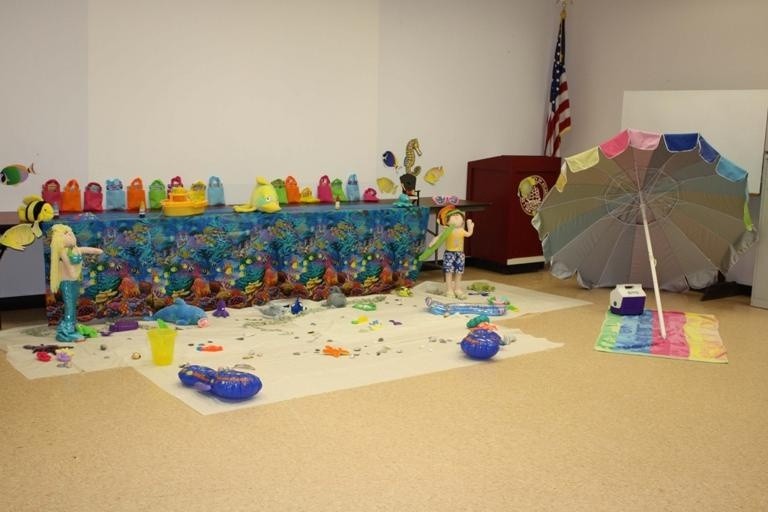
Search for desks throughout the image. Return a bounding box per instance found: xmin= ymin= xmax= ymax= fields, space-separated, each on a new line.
xmin=1 ymin=198 xmax=492 ymax=309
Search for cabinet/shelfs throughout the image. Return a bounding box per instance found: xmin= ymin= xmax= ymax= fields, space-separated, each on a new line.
xmin=464 ymin=154 xmax=561 ymax=267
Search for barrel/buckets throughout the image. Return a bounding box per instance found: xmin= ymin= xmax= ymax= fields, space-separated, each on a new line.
xmin=147 ymin=327 xmax=176 ymax=366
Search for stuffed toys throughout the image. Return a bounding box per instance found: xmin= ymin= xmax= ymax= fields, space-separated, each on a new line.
xmin=234 ymin=175 xmax=282 ymax=213
xmin=144 ymin=297 xmax=208 ymax=327
xmin=324 ymin=286 xmax=347 ymax=308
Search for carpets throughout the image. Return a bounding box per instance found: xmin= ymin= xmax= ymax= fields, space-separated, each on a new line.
xmin=593 ymin=305 xmax=729 ymax=364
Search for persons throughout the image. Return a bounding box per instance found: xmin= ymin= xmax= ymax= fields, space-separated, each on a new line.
xmin=428 ymin=206 xmax=475 ymax=300
xmin=48 ymin=224 xmax=104 ymax=343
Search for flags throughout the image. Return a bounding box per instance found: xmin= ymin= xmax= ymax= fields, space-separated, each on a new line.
xmin=542 ymin=7 xmax=573 ymax=159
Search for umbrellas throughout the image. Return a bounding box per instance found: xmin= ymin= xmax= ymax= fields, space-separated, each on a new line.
xmin=531 ymin=129 xmax=760 ymax=339
